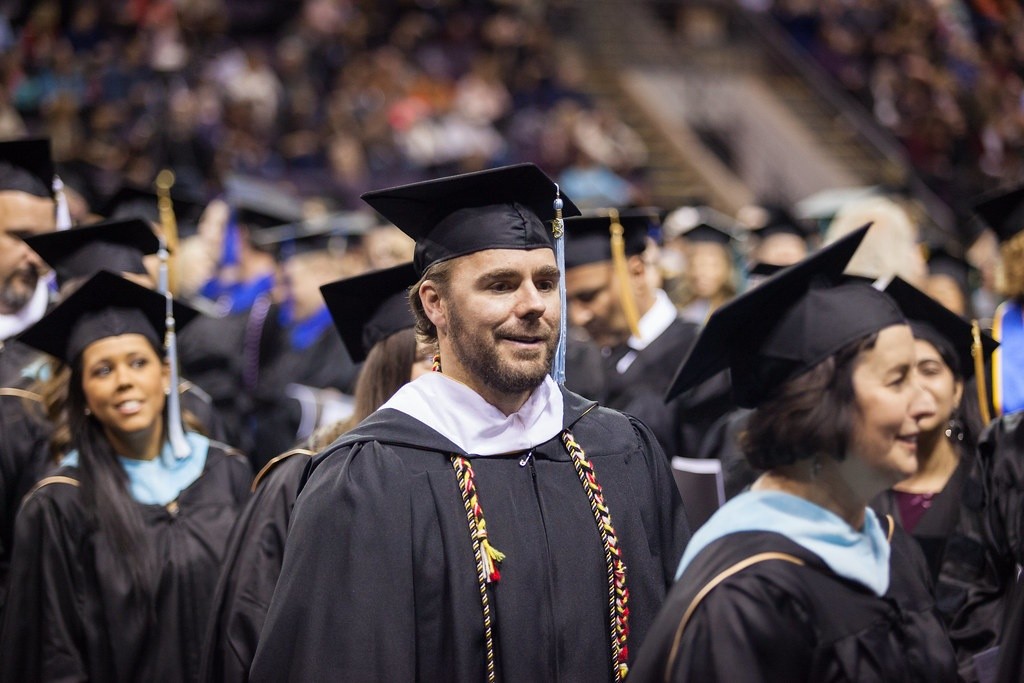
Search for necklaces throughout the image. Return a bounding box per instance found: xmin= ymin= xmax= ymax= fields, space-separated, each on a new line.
xmin=448 ymin=432 xmax=633 ymax=680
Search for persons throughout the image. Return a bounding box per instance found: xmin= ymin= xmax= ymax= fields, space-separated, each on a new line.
xmin=0 ymin=0 xmax=1024 ymax=682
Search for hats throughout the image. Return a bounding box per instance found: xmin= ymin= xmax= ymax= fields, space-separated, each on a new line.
xmin=662 ymin=219 xmax=905 ymax=411
xmin=751 ymin=204 xmax=835 ymax=237
xmin=745 ymin=261 xmax=878 ymax=288
xmin=883 ymin=275 xmax=1001 ymax=428
xmin=966 ymin=180 xmax=1024 ymax=243
xmin=55 ymin=158 xmax=145 ymax=219
xmin=11 ymin=269 xmax=204 ymax=461
xmin=23 ymin=214 xmax=169 ymax=294
xmin=318 ymin=259 xmax=419 ymax=364
xmin=253 ymin=230 xmax=361 ymax=262
xmin=681 ymin=222 xmax=732 ymax=245
xmin=544 ymin=211 xmax=651 ymax=340
xmin=360 ymin=162 xmax=583 ymax=385
xmin=0 ymin=136 xmax=71 ymax=230
